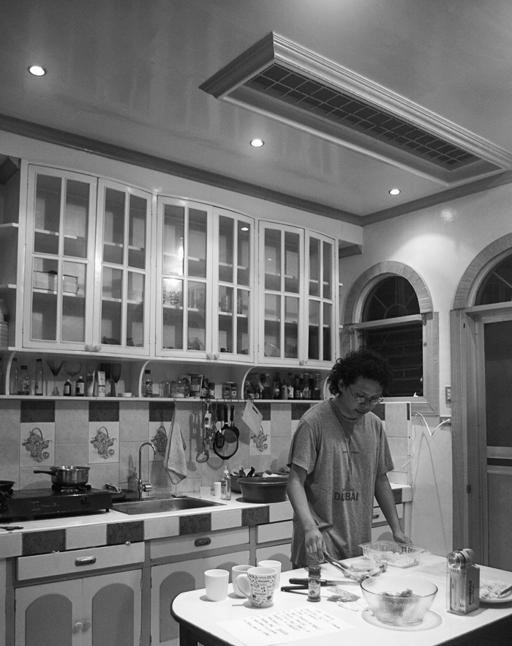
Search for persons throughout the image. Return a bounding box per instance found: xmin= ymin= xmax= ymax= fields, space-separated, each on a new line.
xmin=283 ymin=349 xmax=416 ymax=570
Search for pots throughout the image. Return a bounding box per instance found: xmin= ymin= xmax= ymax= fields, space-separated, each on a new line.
xmin=32 ymin=463 xmax=90 ymax=486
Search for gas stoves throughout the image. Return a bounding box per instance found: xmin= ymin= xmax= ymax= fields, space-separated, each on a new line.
xmin=1 ymin=484 xmax=115 ymax=522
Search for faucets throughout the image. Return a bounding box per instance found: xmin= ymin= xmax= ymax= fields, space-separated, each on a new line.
xmin=138 ymin=440 xmax=158 ymax=500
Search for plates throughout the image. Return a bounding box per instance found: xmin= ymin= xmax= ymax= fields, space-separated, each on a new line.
xmin=360 ymin=608 xmax=442 ymax=634
xmin=476 ymin=583 xmax=510 ymax=607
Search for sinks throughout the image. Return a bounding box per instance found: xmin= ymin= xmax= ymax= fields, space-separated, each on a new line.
xmin=109 ymin=495 xmax=228 ymax=516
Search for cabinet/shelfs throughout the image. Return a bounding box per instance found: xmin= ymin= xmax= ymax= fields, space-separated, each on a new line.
xmin=0 ymin=159 xmax=152 ymax=401
xmin=15 ymin=543 xmax=148 ymax=646
xmin=154 ymin=192 xmax=255 ymax=403
xmin=258 ymin=219 xmax=337 ymax=403
xmin=148 ymin=527 xmax=255 ymax=646
xmin=257 ymin=505 xmax=405 ymax=571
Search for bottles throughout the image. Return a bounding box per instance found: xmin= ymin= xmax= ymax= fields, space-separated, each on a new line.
xmin=143 ymin=369 xmax=239 ymax=400
xmin=306 ymin=565 xmax=321 ymax=601
xmin=10 ymin=356 xmax=134 ymax=398
xmin=200 ymin=468 xmax=232 ymax=502
xmin=245 ymin=370 xmax=323 ymax=400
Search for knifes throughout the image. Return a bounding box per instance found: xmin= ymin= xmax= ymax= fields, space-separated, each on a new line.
xmin=288 ymin=575 xmax=357 ymax=587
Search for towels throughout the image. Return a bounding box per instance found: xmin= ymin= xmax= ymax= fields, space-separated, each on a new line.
xmin=162 ymin=406 xmax=187 ymax=486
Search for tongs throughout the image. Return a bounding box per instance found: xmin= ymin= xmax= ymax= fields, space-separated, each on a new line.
xmin=321 ymin=552 xmax=360 ymax=585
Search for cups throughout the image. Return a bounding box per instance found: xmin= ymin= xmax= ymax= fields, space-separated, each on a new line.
xmin=204 ymin=559 xmax=283 ymax=609
xmin=220 ymin=293 xmax=244 ymax=314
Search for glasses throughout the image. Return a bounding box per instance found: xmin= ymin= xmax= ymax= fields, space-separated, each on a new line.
xmin=349 ymin=384 xmax=384 ymax=404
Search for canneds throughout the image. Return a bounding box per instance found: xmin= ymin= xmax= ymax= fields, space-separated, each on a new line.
xmin=281 ymin=384 xmax=288 ymax=399
xmin=288 ymin=383 xmax=295 ymax=398
xmin=208 ymin=382 xmax=215 ymax=398
xmin=222 ymin=382 xmax=238 ymax=399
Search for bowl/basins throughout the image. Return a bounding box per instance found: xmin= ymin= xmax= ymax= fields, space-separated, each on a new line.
xmin=359 ymin=572 xmax=436 ymax=625
xmin=0 ymin=480 xmax=16 ymax=490
xmin=235 ymin=475 xmax=287 ymax=503
